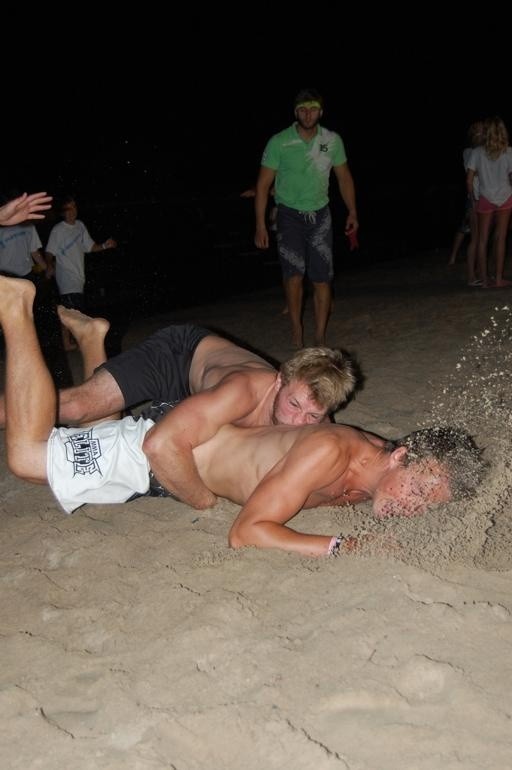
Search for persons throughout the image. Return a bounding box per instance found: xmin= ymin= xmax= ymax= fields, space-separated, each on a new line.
xmin=462 ymin=121 xmax=495 ymax=286
xmin=253 ymin=89 xmax=360 ymax=350
xmin=0 ymin=223 xmax=50 ymax=278
xmin=240 ymin=184 xmax=336 ymax=316
xmin=1 ymin=274 xmax=491 ymax=559
xmin=466 ymin=116 xmax=512 ymax=289
xmin=0 ymin=303 xmax=356 ymax=510
xmin=43 ymin=196 xmax=117 ymax=352
xmin=448 ymin=201 xmax=472 ymax=265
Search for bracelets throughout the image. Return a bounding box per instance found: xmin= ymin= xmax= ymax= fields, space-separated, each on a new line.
xmin=102 ymin=243 xmax=106 ymax=250
xmin=327 ymin=533 xmax=356 ymax=560
